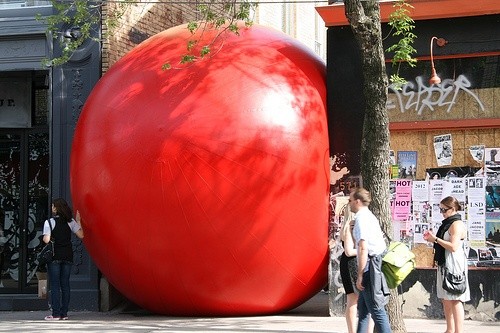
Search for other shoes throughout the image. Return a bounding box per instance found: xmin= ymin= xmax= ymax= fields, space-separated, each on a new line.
xmin=62 ymin=316 xmax=69 ymax=319
xmin=45 ymin=315 xmax=61 ymax=320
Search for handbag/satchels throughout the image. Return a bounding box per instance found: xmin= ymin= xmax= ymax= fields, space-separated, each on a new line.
xmin=37 ymin=240 xmax=55 ymax=263
xmin=442 ymin=271 xmax=466 ymax=293
xmin=382 ymin=241 xmax=416 ymax=289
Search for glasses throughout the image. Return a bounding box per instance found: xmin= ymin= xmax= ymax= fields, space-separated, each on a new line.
xmin=349 ymin=199 xmax=356 ymax=202
xmin=440 ymin=208 xmax=452 ymax=213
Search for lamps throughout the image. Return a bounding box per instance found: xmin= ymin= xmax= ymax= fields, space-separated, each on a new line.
xmin=428 ymin=37 xmax=446 ymax=84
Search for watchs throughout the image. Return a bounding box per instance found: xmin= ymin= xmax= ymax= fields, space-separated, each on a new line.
xmin=434 ymin=237 xmax=438 ymax=244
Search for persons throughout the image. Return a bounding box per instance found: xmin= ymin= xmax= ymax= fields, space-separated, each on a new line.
xmin=340 ymin=202 xmax=359 ymax=333
xmin=349 ymin=187 xmax=392 ymax=333
xmin=423 ymin=196 xmax=471 ymax=333
xmin=42 ymin=198 xmax=84 ymax=321
